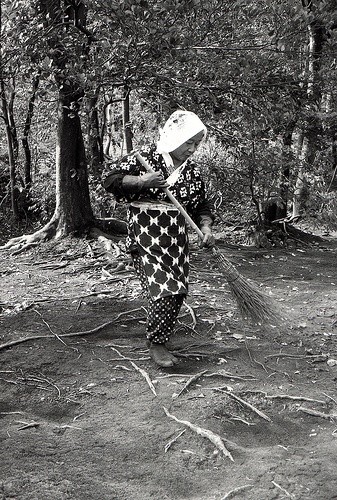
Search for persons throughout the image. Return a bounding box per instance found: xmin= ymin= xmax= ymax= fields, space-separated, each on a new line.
xmin=102 ymin=111 xmax=215 ymax=368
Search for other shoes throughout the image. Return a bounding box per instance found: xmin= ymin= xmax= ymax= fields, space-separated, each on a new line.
xmin=148 ymin=342 xmax=174 ymax=368
xmin=145 ymin=340 xmax=178 ymax=363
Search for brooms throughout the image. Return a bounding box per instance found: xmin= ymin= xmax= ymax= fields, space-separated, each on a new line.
xmin=132 ymin=150 xmax=287 ymax=336
